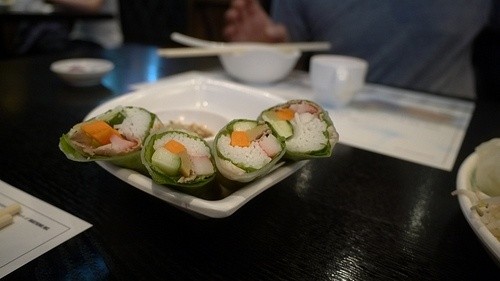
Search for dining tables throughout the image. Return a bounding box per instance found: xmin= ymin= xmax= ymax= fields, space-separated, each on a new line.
xmin=1 ymin=0 xmax=500 ymax=280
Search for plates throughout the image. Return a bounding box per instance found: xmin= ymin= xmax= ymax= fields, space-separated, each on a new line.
xmin=78 ymin=71 xmax=324 ymax=217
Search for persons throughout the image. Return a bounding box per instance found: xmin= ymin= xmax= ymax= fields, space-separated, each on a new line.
xmin=223 ymin=0 xmax=495 ymax=96
xmin=0 ymin=0 xmax=124 ymax=65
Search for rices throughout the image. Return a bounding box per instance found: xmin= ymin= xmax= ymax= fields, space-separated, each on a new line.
xmin=59 ymin=99 xmax=340 ymax=190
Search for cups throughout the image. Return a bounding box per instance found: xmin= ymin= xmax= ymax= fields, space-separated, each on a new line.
xmin=308 ymin=54 xmax=370 ymax=110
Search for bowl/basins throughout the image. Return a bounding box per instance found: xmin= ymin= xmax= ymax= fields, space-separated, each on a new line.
xmin=50 ymin=57 xmax=118 ymax=90
xmin=456 ymin=133 xmax=499 ymax=263
xmin=216 ymin=48 xmax=302 ymax=85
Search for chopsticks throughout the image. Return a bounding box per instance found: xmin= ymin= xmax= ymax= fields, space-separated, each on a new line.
xmin=157 ymin=43 xmax=331 ymax=60
